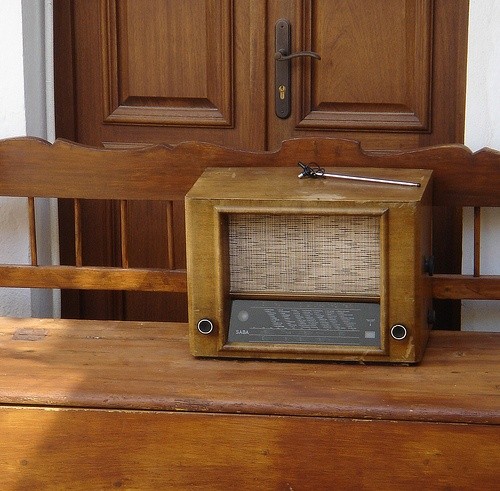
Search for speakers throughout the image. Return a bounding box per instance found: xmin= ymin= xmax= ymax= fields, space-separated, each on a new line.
xmin=185 ymin=166 xmax=434 ymax=363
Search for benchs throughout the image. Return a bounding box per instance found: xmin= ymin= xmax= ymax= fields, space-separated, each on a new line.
xmin=0 ymin=133 xmax=500 ymax=491
